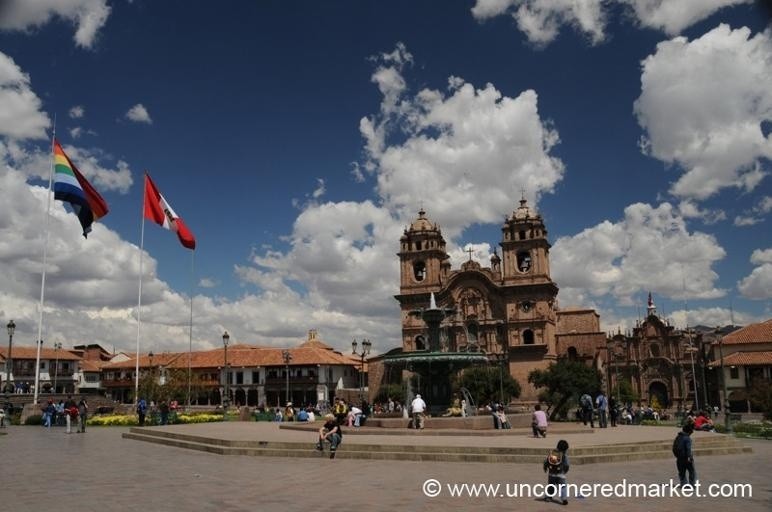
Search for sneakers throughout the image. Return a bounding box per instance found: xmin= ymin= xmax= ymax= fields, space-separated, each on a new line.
xmin=534 ymin=432 xmax=547 ymax=438
xmin=584 ymin=424 xmax=618 ymax=428
xmin=45 ymin=424 xmax=86 ymax=433
xmin=563 ymin=500 xmax=568 ymax=505
xmin=545 ymin=497 xmax=553 ymax=502
xmin=316 ymin=446 xmax=337 ymax=452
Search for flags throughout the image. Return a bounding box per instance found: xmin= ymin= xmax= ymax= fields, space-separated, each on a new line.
xmin=51 ymin=138 xmax=110 ymax=240
xmin=142 ymin=169 xmax=197 ymax=251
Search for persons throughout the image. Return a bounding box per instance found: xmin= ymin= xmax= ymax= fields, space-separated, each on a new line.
xmin=1 ymin=394 xmax=90 ymax=434
xmin=254 ymin=397 xmax=402 ymax=428
xmin=441 ymin=392 xmax=462 ymax=417
xmin=411 ymin=393 xmax=427 ymax=429
xmin=680 ymin=403 xmax=720 ymax=433
xmin=137 ymin=397 xmax=148 ymax=424
xmin=150 ymin=398 xmax=158 ymax=425
xmin=160 ymin=399 xmax=170 ymax=425
xmin=495 ymin=405 xmax=509 ymax=429
xmin=531 ymin=404 xmax=547 ymax=438
xmin=315 ymin=413 xmax=343 ymax=453
xmin=15 ymin=380 xmax=32 ymax=395
xmin=576 ymin=387 xmax=670 ymax=428
xmin=673 ymin=425 xmax=697 ymax=490
xmin=542 ymin=439 xmax=570 ymax=505
xmin=170 ymin=399 xmax=179 ymax=424
xmin=486 ymin=395 xmax=512 ymax=429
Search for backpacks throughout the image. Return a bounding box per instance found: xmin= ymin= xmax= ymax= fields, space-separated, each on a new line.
xmin=548 ymin=450 xmax=563 ymax=473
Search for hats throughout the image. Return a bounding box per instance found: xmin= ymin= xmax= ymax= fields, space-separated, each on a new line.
xmin=557 ymin=440 xmax=568 ymax=450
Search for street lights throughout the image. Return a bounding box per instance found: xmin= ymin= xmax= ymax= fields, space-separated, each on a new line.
xmin=714 ymin=325 xmax=732 ymax=433
xmin=223 ymin=329 xmax=231 ymax=408
xmin=496 ymin=348 xmax=510 ymax=402
xmin=698 ymin=357 xmax=710 ymax=411
xmin=351 ymin=337 xmax=371 ymax=404
xmin=282 ymin=347 xmax=292 ymax=407
xmin=686 ymin=347 xmax=700 ymax=411
xmin=4 ymin=318 xmax=17 ymax=392
xmin=148 ymin=350 xmax=154 ymax=374
xmin=54 ymin=341 xmax=63 ymax=391
xmin=612 ymin=352 xmax=622 ymax=405
